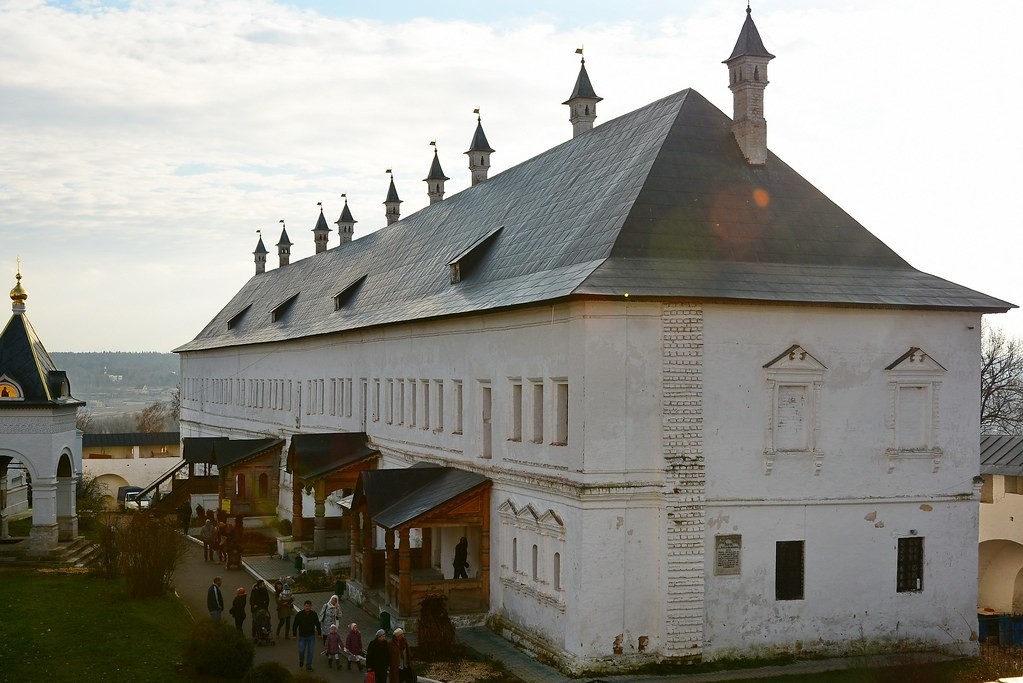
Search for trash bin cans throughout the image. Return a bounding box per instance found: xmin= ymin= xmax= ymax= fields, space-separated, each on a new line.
xmin=335 ymin=581 xmax=344 ymax=595
xmin=294 ymin=556 xmax=302 ymax=569
xmin=380 ymin=612 xmax=390 ymax=630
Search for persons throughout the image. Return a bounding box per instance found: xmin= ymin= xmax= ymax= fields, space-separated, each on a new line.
xmin=183 ymin=501 xmax=413 ymax=683
xmin=452 ymin=537 xmax=469 ymax=580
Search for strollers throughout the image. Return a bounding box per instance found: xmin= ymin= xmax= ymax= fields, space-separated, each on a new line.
xmin=252 ymin=605 xmax=275 ymax=648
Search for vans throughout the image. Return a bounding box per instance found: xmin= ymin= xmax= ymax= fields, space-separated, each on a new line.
xmin=149 ymin=491 xmax=170 ymax=510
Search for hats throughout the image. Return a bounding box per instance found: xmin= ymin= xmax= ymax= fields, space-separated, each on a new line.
xmin=283 ymin=583 xmax=289 ymax=589
xmin=347 ymin=623 xmax=356 ymax=630
xmin=375 ymin=629 xmax=385 ymax=636
xmin=392 ymin=628 xmax=402 ymax=636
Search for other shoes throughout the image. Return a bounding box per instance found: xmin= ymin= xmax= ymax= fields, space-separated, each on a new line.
xmin=285 ymin=637 xmax=291 ymax=640
xmin=306 ymin=668 xmax=312 ymax=671
xmin=275 ymin=633 xmax=279 ymax=638
xmin=299 ymin=661 xmax=303 ymax=666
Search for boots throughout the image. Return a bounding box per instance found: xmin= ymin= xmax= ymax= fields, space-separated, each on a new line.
xmin=357 ymin=660 xmax=364 ymax=671
xmin=347 ymin=660 xmax=352 ymax=669
xmin=328 ymin=658 xmax=332 ymax=667
xmin=336 ymin=658 xmax=342 ymax=669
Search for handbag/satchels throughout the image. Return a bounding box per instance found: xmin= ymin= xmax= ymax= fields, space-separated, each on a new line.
xmin=363 ymin=671 xmax=375 ymax=683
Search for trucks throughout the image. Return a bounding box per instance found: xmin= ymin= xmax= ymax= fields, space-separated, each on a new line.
xmin=116 ymin=486 xmax=150 ymax=515
